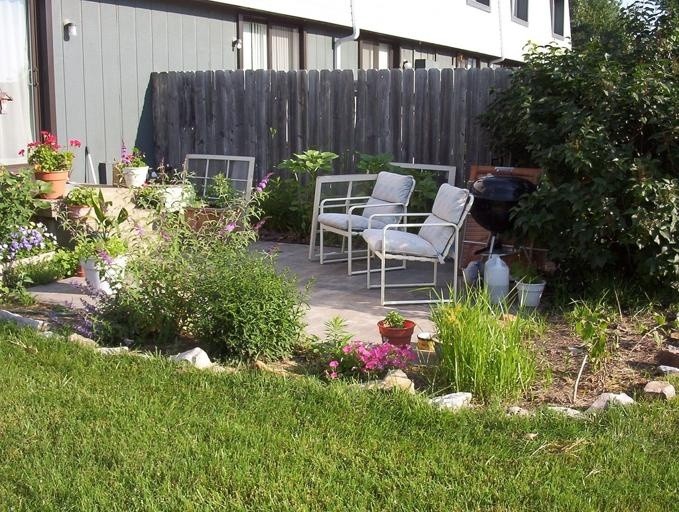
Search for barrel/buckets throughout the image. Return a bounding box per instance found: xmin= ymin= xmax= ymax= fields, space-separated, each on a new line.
xmin=484 ymin=254 xmax=510 ymax=305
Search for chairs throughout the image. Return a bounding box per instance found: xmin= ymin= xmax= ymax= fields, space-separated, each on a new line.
xmin=317 ymin=171 xmax=417 ymax=276
xmin=361 ymin=182 xmax=474 ymax=306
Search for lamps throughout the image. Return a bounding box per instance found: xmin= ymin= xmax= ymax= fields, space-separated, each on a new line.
xmin=403 ymin=60 xmax=412 ymax=71
xmin=63 ymin=19 xmax=76 ymax=36
xmin=232 ymin=36 xmax=241 ymax=49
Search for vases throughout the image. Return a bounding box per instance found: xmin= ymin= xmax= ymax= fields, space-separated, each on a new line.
xmin=35 ymin=170 xmax=68 ymax=199
xmin=124 ymin=166 xmax=148 ymax=187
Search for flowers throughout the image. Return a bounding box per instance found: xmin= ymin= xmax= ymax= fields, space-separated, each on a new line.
xmin=18 ymin=133 xmax=81 ymax=171
xmin=119 ymin=141 xmax=147 ymax=165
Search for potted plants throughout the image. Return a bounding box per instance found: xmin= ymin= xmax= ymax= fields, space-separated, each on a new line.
xmin=511 ymin=261 xmax=547 ymax=307
xmin=78 ymin=189 xmax=130 ymax=296
xmin=378 ymin=313 xmax=415 ymax=346
xmin=64 ymin=185 xmax=96 ymax=221
